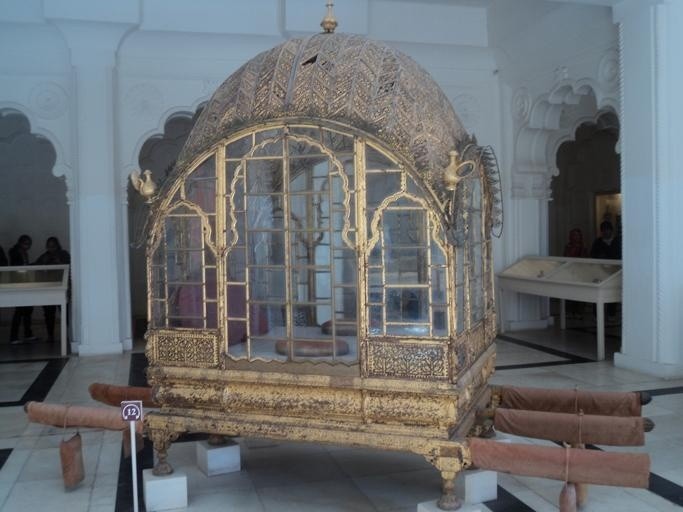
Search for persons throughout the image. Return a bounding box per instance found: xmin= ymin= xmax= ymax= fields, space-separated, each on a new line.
xmin=8 ymin=235 xmax=38 ymax=345
xmin=36 ymin=237 xmax=71 ymax=344
xmin=564 ymin=229 xmax=588 ymax=320
xmin=590 ymin=221 xmax=620 ymax=322
